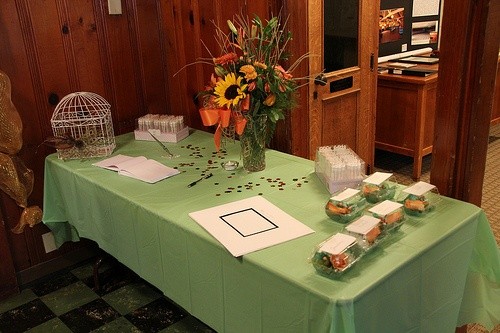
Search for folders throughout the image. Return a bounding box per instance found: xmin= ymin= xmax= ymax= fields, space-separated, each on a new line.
xmin=388 ymin=68 xmax=438 ymax=77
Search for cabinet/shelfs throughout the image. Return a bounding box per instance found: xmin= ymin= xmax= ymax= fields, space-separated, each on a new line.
xmin=375 ymin=50 xmax=500 ymax=182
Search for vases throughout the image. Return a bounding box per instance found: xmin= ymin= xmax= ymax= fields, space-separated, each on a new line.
xmin=239 ymin=114 xmax=267 ymax=172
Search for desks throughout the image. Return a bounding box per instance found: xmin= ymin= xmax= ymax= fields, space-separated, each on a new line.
xmin=41 ymin=129 xmax=500 ymax=333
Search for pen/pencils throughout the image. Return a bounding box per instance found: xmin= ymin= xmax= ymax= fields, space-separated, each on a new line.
xmin=188 ymin=173 xmax=213 ymax=187
xmin=148 ymin=130 xmax=173 ymax=157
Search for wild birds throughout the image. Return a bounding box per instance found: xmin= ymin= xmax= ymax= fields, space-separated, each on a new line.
xmin=35 ymin=132 xmax=84 ymax=153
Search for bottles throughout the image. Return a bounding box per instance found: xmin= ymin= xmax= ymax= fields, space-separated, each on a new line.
xmin=429 ymin=31 xmax=437 ymax=43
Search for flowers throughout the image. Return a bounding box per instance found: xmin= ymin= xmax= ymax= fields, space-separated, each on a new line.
xmin=172 ymin=0 xmax=327 ymax=153
